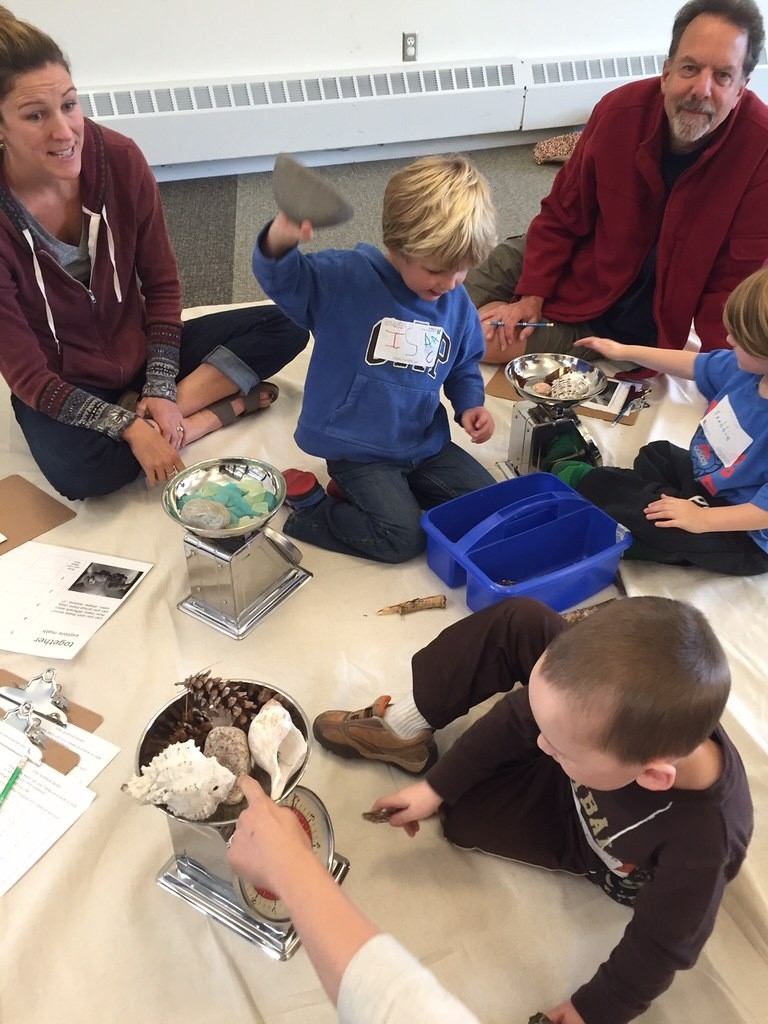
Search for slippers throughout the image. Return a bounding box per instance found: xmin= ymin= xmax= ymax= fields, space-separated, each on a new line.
xmin=203 ymin=382 xmax=279 ymax=428
xmin=119 ymin=388 xmax=142 ymax=415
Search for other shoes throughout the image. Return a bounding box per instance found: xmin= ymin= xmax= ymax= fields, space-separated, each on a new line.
xmin=281 ymin=468 xmax=351 ymax=511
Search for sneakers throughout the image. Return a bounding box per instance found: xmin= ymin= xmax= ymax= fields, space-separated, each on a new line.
xmin=313 ymin=695 xmax=439 ymax=777
xmin=552 ymin=460 xmax=596 ymax=491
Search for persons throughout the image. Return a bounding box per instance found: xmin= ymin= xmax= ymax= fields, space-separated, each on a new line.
xmin=0 ymin=5 xmax=310 ymax=502
xmin=252 ymin=151 xmax=499 ymax=565
xmin=313 ymin=597 xmax=754 ymax=1023
xmin=553 ymin=269 xmax=767 ymax=577
xmin=227 ymin=772 xmax=479 ymax=1024
xmin=460 ymin=0 xmax=768 ymax=365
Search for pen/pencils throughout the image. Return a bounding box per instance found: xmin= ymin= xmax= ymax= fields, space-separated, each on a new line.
xmin=610 ymin=400 xmax=638 ymax=428
xmin=0 ymin=757 xmax=28 ymax=812
xmin=485 ymin=322 xmax=554 ymax=327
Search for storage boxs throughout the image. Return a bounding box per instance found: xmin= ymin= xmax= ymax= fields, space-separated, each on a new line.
xmin=420 ymin=471 xmax=635 ymax=617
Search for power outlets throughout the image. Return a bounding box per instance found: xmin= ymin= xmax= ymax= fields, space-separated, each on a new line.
xmin=403 ymin=32 xmax=419 ymax=62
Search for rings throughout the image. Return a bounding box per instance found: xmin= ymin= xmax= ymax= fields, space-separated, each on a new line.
xmin=177 ymin=426 xmax=184 ymax=432
xmin=165 ymin=468 xmax=176 ymax=476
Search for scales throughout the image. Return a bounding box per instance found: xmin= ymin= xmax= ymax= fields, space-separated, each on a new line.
xmin=161 ymin=457 xmax=313 ymax=640
xmin=496 ymin=353 xmax=608 ymax=480
xmin=133 ymin=676 xmax=352 ymax=963
